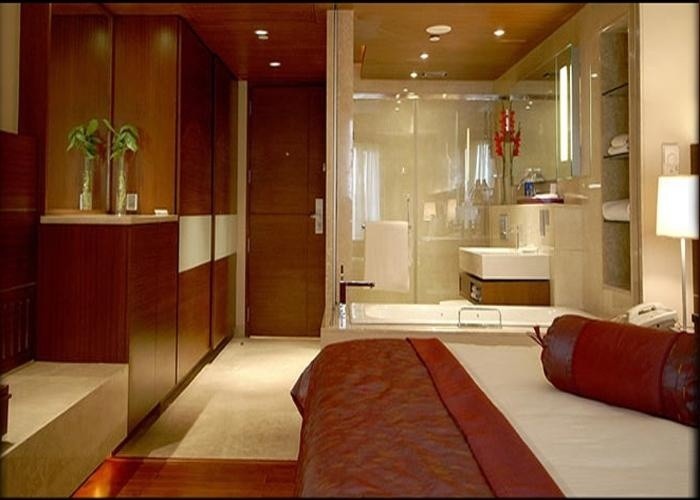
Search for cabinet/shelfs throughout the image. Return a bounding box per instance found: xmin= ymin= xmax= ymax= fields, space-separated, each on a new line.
xmin=597 ymin=16 xmax=632 ymax=293
xmin=39 ymin=220 xmax=178 ymax=436
xmin=111 ymin=12 xmax=239 ymax=384
xmin=457 ymin=273 xmax=550 ymax=306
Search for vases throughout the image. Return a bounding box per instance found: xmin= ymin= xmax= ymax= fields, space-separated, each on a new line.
xmin=492 ymin=175 xmax=506 ymax=205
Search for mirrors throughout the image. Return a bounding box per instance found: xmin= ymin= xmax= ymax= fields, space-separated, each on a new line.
xmin=510 ymin=41 xmax=576 ymax=186
xmin=46 ymin=0 xmax=113 ymax=216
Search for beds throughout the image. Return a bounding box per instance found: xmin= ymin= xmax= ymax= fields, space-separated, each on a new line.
xmin=299 ymin=336 xmax=699 ymax=498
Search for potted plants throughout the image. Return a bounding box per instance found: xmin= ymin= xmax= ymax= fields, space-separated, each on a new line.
xmin=103 ymin=119 xmax=141 ymax=219
xmin=65 ymin=117 xmax=103 ymax=211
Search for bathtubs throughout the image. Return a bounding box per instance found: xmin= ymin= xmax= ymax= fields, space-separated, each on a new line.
xmin=351 ymin=304 xmax=598 ymax=326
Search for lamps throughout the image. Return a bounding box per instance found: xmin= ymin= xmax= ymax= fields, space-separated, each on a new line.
xmin=654 ymin=174 xmax=699 ymax=334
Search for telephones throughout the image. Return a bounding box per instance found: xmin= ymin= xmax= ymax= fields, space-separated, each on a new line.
xmin=620 ymin=303 xmax=678 ymax=327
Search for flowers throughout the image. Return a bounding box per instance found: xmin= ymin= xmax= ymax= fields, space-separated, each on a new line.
xmin=492 ymin=104 xmax=511 ymax=203
xmin=511 ymin=111 xmax=523 ymax=187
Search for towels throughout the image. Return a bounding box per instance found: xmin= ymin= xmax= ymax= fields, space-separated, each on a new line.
xmin=363 ymin=219 xmax=411 ymax=294
xmin=602 ymin=198 xmax=630 ymax=223
xmin=604 ymin=135 xmax=629 ymax=156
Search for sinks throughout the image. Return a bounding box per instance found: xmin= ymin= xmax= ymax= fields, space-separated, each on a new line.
xmin=458 ymin=246 xmax=548 ymax=281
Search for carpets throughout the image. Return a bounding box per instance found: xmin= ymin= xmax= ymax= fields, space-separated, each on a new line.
xmin=116 ymin=335 xmax=319 ymax=461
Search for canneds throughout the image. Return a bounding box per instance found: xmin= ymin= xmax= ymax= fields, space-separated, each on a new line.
xmin=524 ymin=182 xmax=534 ymax=197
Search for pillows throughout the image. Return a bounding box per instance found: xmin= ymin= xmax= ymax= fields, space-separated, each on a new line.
xmin=523 ymin=313 xmax=699 ymax=431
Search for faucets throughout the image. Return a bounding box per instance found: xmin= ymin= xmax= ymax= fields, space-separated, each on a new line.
xmin=502 ymin=224 xmax=520 ymax=248
xmin=338 ymin=279 xmax=375 ymax=303
